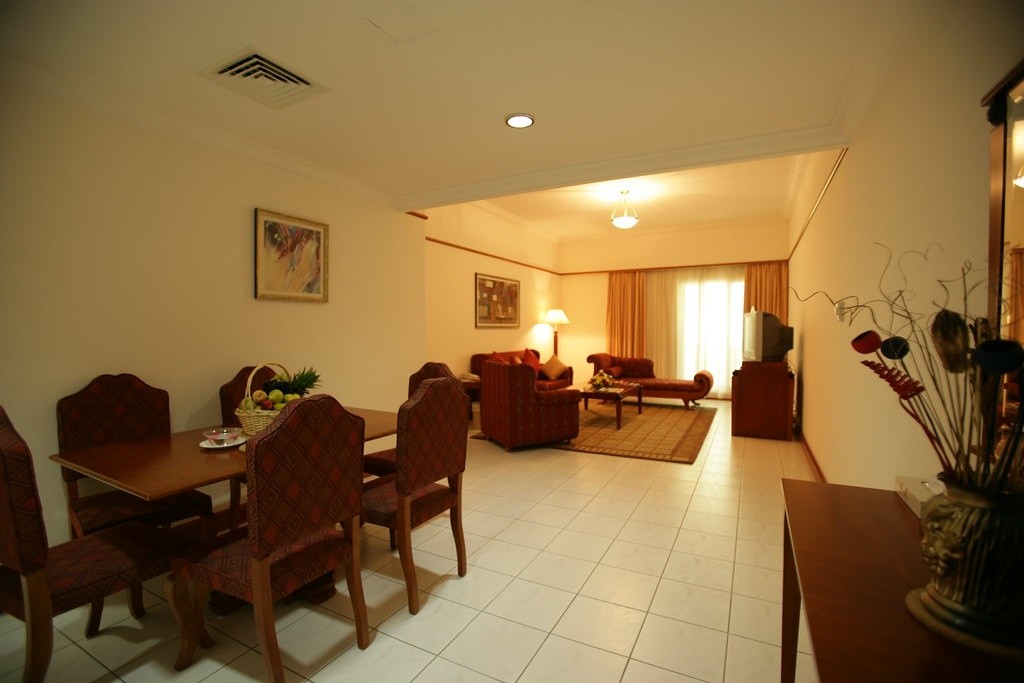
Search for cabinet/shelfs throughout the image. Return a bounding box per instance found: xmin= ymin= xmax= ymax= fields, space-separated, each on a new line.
xmin=731 ymin=362 xmax=796 ymax=442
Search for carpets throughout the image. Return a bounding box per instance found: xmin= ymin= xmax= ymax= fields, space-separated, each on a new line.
xmin=469 ymin=401 xmax=717 ymax=465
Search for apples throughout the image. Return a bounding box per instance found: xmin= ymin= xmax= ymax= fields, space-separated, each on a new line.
xmin=252 ymin=390 xmax=267 ymax=404
xmin=260 ymin=399 xmax=272 ymax=410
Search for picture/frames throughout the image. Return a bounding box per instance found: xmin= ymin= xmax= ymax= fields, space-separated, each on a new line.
xmin=475 ymin=272 xmax=521 ymax=330
xmin=254 ymin=205 xmax=331 ymax=303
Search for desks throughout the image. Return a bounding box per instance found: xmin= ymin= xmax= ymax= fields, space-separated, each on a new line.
xmin=782 ymin=478 xmax=1023 ymax=683
xmin=47 ymin=405 xmax=397 ymax=508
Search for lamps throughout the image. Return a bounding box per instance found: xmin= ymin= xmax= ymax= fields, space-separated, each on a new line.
xmin=545 ymin=309 xmax=570 ymax=358
xmin=611 ymin=185 xmax=640 ymax=231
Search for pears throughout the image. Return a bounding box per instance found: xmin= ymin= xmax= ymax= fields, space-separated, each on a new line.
xmin=240 ymin=389 xmax=300 ymax=411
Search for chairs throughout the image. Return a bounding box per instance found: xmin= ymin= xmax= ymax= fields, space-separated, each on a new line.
xmin=365 ymin=378 xmax=469 ymax=616
xmin=170 ymin=393 xmax=368 ymax=682
xmin=57 ymin=373 xmax=213 ymax=537
xmin=363 ymin=361 xmax=462 ymax=550
xmin=0 ymin=406 xmax=215 ymax=683
xmin=219 ymin=364 xmax=278 ymax=427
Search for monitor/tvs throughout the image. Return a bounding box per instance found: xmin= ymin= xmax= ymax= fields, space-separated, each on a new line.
xmin=742 ymin=311 xmax=794 ymax=361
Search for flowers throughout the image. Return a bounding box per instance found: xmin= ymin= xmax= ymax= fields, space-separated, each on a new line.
xmin=588 ymin=370 xmax=614 ymax=389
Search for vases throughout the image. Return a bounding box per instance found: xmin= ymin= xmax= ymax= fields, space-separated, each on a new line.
xmin=903 ymin=469 xmax=1023 ymax=661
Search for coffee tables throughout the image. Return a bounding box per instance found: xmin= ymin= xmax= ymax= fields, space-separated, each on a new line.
xmin=563 ymin=380 xmax=643 ymax=429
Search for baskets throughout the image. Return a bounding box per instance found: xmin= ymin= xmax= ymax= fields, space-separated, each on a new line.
xmin=234 ymin=362 xmax=292 ymax=436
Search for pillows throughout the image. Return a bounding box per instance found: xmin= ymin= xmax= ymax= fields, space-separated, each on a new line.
xmin=607 ymin=365 xmax=625 ymax=378
xmin=491 ymin=349 xmax=569 ymax=381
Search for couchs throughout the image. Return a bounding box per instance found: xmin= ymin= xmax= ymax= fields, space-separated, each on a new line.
xmin=472 ymin=350 xmax=573 ymax=390
xmin=479 ymin=359 xmax=581 ymax=452
xmin=588 ymin=354 xmax=713 ymax=410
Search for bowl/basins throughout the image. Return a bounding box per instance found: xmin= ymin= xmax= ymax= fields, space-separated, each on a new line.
xmin=202 ymin=427 xmax=243 ymax=446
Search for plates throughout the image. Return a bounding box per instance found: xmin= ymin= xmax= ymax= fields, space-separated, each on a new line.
xmin=199 ymin=437 xmax=247 ymax=449
